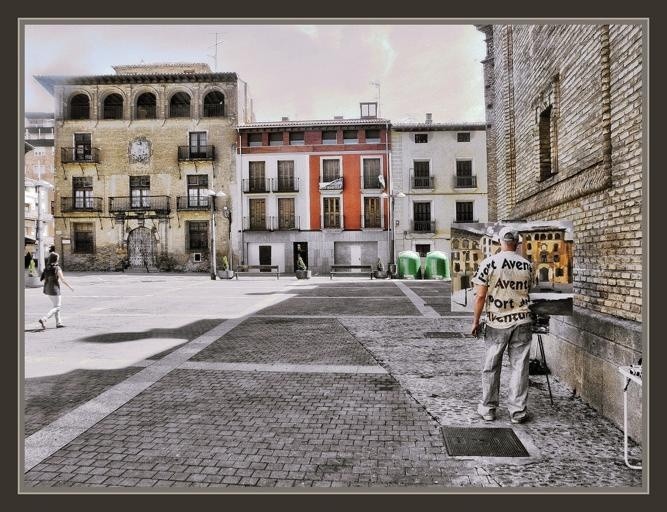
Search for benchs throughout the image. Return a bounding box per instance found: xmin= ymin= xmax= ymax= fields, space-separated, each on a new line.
xmin=329 ymin=265 xmax=374 ymax=279
xmin=234 ymin=264 xmax=280 ymax=280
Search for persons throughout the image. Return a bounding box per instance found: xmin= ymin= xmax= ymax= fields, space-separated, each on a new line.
xmin=39 ymin=251 xmax=75 ymax=328
xmin=472 ymin=227 xmax=535 ymax=423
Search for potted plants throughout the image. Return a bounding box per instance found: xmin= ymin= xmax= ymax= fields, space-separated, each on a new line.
xmin=374 ymin=257 xmax=388 ymax=278
xmin=296 ymin=257 xmax=311 ymax=279
xmin=217 ymin=255 xmax=234 ymax=279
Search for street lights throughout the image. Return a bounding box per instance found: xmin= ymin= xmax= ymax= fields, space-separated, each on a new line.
xmin=32 ymin=181 xmax=50 ymax=275
xmin=202 ymin=189 xmax=227 ymax=280
xmin=381 ymin=190 xmax=406 ymax=264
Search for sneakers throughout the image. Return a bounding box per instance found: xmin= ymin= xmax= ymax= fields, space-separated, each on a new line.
xmin=56 ymin=326 xmax=64 ymax=328
xmin=39 ymin=319 xmax=47 ymax=330
xmin=476 ymin=403 xmax=494 ymax=421
xmin=510 ymin=411 xmax=534 ymax=424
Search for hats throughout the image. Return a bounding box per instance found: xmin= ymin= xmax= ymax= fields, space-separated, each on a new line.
xmin=498 ymin=226 xmax=519 ymax=242
